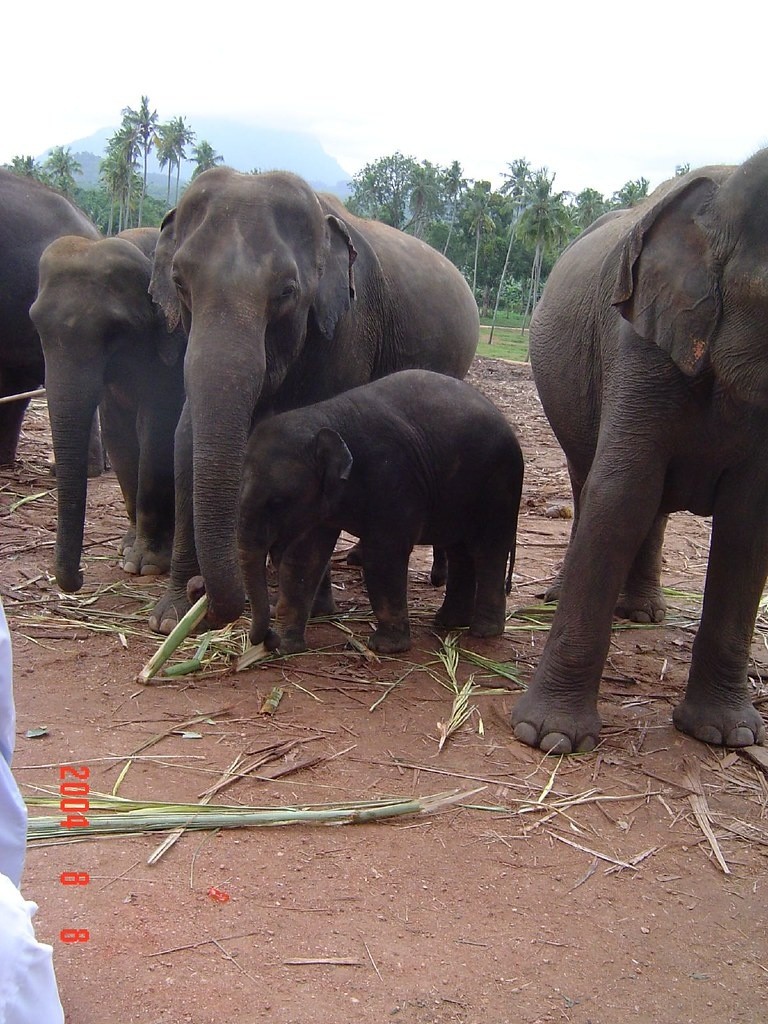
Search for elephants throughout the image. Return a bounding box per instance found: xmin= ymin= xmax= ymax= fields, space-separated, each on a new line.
xmin=1 ymin=146 xmax=768 ymax=759
